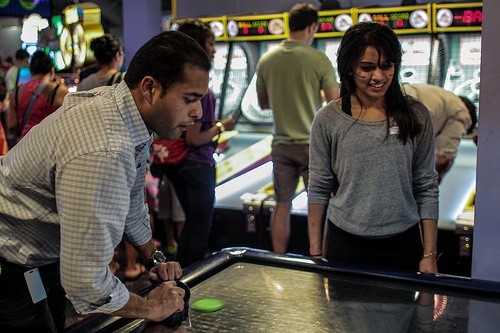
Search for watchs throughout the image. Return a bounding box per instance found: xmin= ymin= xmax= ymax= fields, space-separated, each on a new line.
xmin=145 ymin=250 xmax=167 ymax=270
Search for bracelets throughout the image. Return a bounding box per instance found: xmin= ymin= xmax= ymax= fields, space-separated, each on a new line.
xmin=309 ymin=253 xmax=322 ymax=256
xmin=423 ymin=252 xmax=437 ymax=257
xmin=215 ymin=121 xmax=225 ymax=134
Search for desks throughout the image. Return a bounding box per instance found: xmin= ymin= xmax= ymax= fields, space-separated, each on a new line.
xmin=75 ymin=246 xmax=500 ymax=333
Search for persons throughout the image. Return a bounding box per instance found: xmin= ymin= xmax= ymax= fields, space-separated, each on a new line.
xmin=399 ymin=83 xmax=477 ymax=182
xmin=8 ymin=50 xmax=70 ymax=146
xmin=308 ymin=21 xmax=440 ymax=308
xmin=255 ymin=3 xmax=341 ymax=261
xmin=0 ymin=75 xmax=10 ymax=156
xmin=0 ymin=31 xmax=212 ymax=333
xmin=3 ymin=48 xmax=29 ymax=91
xmin=76 ymin=34 xmax=127 ymax=91
xmin=1 ymin=55 xmax=13 ymax=70
xmin=156 ymin=19 xmax=237 ymax=271
